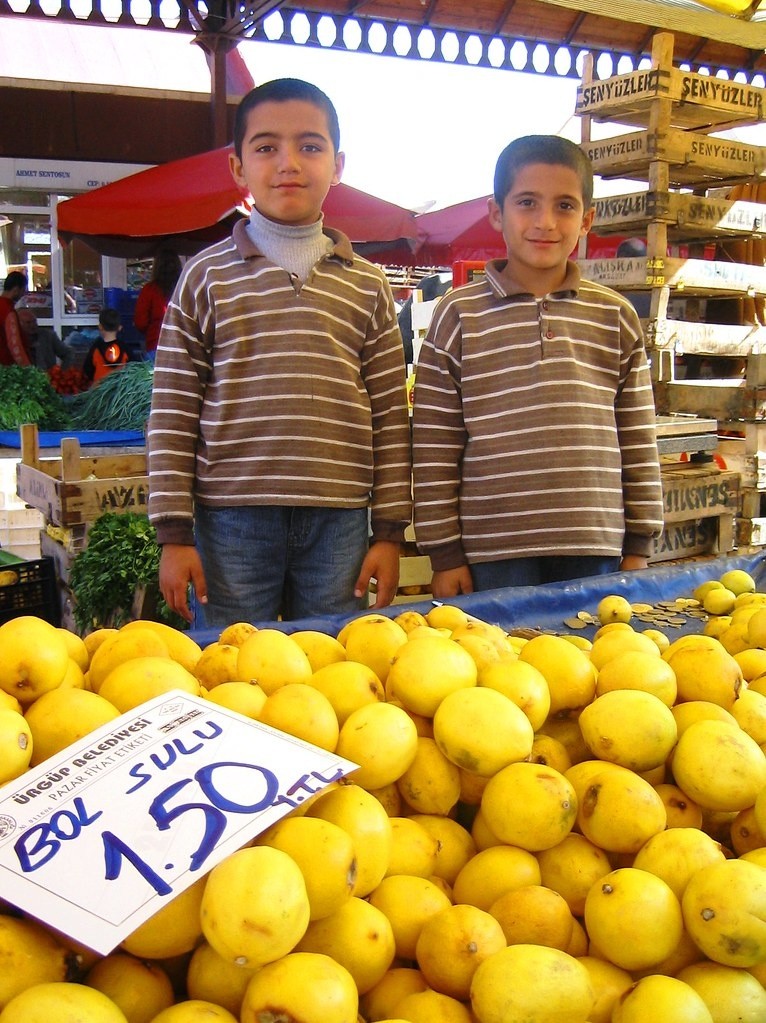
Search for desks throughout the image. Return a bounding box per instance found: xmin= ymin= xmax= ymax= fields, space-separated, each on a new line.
xmin=175 ymin=552 xmax=766 ymax=646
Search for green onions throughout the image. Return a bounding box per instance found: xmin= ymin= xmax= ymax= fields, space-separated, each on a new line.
xmin=34 ymin=356 xmax=152 ymax=435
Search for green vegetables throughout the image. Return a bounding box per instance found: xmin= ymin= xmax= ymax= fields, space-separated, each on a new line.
xmin=65 ymin=512 xmax=194 ymax=635
xmin=0 ymin=362 xmax=72 ymax=431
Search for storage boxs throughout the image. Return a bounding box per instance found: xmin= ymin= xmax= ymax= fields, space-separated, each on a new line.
xmin=0 ymin=556 xmax=62 ymax=628
xmin=71 ymin=287 xmax=104 ymax=313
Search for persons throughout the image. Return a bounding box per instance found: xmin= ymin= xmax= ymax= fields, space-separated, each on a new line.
xmin=415 ymin=133 xmax=663 ymax=597
xmin=135 ymin=247 xmax=184 ymax=366
xmin=1 ymin=270 xmax=76 ymax=371
xmin=146 ymin=77 xmax=412 ymax=626
xmin=610 ymin=237 xmax=651 ymax=319
xmin=82 ymin=308 xmax=135 ymax=388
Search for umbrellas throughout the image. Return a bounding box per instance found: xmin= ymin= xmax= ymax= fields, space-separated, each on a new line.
xmin=356 ymin=193 xmax=719 ymax=270
xmin=56 ymin=136 xmax=415 ymax=272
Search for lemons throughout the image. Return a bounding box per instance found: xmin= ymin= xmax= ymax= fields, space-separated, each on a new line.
xmin=0 ymin=570 xmax=766 ymax=1023
